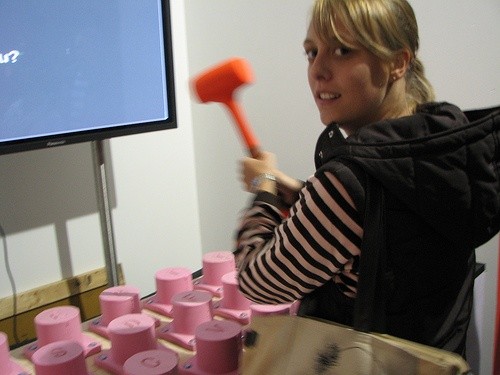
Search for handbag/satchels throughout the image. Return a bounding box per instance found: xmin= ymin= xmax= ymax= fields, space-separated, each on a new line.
xmin=240 ymin=158 xmax=471 ymax=375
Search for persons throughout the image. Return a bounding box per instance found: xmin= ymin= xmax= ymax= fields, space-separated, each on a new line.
xmin=231 ymin=0 xmax=500 ymax=362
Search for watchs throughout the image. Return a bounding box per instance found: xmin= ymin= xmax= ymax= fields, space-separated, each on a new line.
xmin=247 ymin=172 xmax=277 ymax=192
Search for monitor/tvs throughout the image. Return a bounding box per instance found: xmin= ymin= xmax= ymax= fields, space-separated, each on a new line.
xmin=0 ymin=0 xmax=178 ymax=157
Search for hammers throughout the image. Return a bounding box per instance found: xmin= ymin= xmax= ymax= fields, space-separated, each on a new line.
xmin=194 ymin=58 xmax=261 ymax=160
